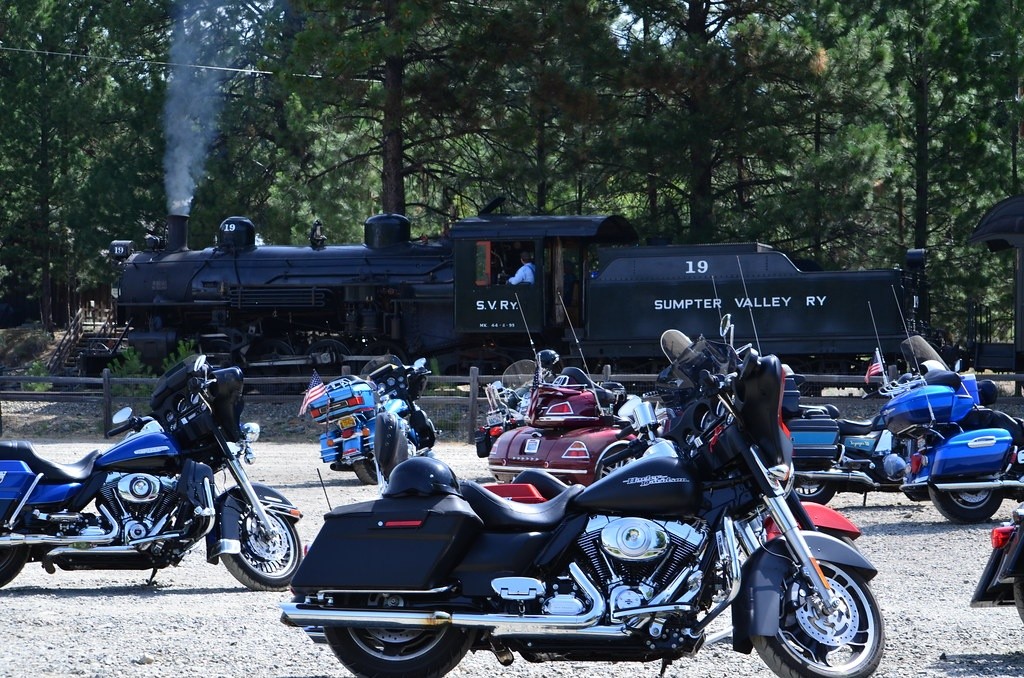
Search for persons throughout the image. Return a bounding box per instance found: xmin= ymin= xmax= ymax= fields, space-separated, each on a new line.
xmin=505 ymin=249 xmax=535 ymax=285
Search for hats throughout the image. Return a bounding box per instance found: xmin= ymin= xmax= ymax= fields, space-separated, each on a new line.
xmin=520 ymin=251 xmax=531 ymax=260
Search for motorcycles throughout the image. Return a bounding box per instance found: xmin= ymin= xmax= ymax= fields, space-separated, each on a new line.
xmin=299 ymin=320 xmax=1024 ymax=678
xmin=1 ymin=312 xmax=304 ymax=593
xmin=302 ymin=349 xmax=440 ymax=480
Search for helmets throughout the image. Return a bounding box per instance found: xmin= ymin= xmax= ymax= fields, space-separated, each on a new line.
xmin=381 ymin=455 xmax=465 ymax=498
xmin=536 ymin=349 xmax=563 ymax=375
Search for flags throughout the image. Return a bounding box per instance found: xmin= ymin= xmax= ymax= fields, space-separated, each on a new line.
xmin=297 ymin=370 xmax=327 ymax=418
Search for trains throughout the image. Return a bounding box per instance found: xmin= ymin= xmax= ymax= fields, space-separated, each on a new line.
xmin=106 ymin=196 xmax=933 ymax=375
